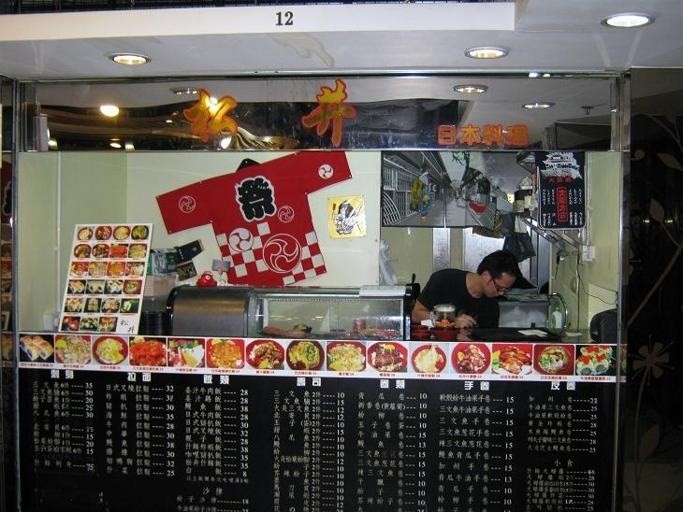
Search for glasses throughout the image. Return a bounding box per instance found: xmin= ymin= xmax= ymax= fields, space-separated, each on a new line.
xmin=491 ymin=279 xmax=511 ymax=293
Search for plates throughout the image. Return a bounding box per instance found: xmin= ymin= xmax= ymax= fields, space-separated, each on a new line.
xmin=113 ymin=223 xmax=151 ymax=244
xmin=166 ymin=336 xmax=206 ymax=370
xmin=448 ymin=342 xmax=491 ymax=375
xmin=407 ymin=341 xmax=449 ymax=374
xmin=491 ymin=344 xmax=534 ymax=375
xmin=128 ymin=335 xmax=167 ymax=369
xmin=245 ymin=338 xmax=286 ymax=372
xmin=366 ymin=341 xmax=408 ymax=373
xmin=74 ymin=225 xmax=111 ymax=259
xmin=205 ymin=337 xmax=245 ymax=371
xmin=91 ymin=335 xmax=129 ymax=367
xmin=325 ymin=341 xmax=370 ymax=374
xmin=54 ymin=334 xmax=92 ymax=365
xmin=533 ymin=344 xmax=574 ymax=376
xmin=285 ymin=340 xmax=327 ymax=372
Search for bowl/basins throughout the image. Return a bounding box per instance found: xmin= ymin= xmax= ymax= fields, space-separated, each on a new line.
xmin=430 ymin=327 xmax=460 ymax=341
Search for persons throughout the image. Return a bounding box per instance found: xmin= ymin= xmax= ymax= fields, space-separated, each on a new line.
xmin=411 ymin=248 xmax=522 ymax=328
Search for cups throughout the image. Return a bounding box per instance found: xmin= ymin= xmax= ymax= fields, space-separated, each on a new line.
xmin=410 ymin=178 xmax=431 ymax=215
xmin=430 ymin=305 xmax=456 ymax=326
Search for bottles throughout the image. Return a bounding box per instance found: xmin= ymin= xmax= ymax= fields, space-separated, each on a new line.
xmin=551 ymin=306 xmax=565 ymax=335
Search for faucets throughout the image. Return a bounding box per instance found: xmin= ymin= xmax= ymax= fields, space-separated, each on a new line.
xmin=551 ymin=292 xmax=568 ymax=333
